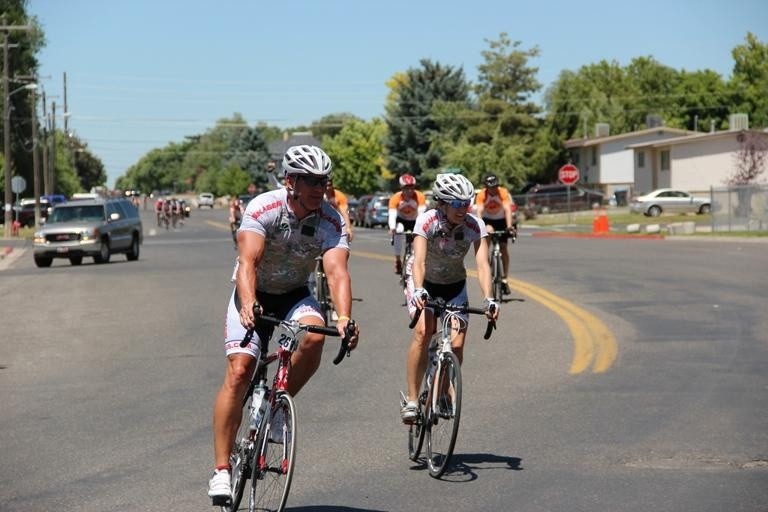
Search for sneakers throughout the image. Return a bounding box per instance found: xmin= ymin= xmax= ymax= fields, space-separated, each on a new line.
xmin=395 ymin=260 xmax=400 ymax=273
xmin=403 ymin=400 xmax=418 ymax=421
xmin=269 ymin=408 xmax=289 ymax=444
xmin=502 ymin=279 xmax=510 ymax=295
xmin=208 ymin=467 xmax=232 ymax=498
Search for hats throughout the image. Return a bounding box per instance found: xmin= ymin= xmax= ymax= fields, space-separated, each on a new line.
xmin=484 ymin=174 xmax=499 ymax=188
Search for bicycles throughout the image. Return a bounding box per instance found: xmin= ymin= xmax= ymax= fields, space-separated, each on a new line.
xmin=484 ymin=229 xmax=515 ymax=303
xmin=399 ymin=292 xmax=497 ymax=479
xmin=389 ymin=230 xmax=417 ymax=297
xmin=308 ymin=253 xmax=336 ymax=326
xmin=217 ymin=302 xmax=357 ymax=512
xmin=229 ymin=213 xmax=243 ymax=250
xmin=156 ymin=206 xmax=184 ymax=230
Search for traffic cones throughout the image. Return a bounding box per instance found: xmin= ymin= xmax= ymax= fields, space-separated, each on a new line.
xmin=592 ymin=204 xmax=599 ymax=232
xmin=597 ymin=205 xmax=609 ymax=232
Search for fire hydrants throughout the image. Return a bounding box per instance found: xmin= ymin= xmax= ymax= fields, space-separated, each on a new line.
xmin=11 ymin=221 xmax=21 ymax=237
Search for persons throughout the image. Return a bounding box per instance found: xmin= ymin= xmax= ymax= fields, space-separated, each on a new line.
xmin=204 ymin=141 xmax=361 ymax=504
xmin=396 ymin=173 xmax=500 ymax=423
xmin=129 ymin=189 xmax=190 ymax=232
xmin=306 ymin=177 xmax=354 ymax=322
xmin=385 ymin=175 xmax=427 ymax=272
xmin=227 ymin=193 xmax=249 ymax=249
xmin=475 ymin=176 xmax=517 ymax=295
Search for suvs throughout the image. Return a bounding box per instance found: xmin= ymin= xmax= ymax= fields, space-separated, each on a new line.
xmin=31 ymin=198 xmax=144 ymax=265
xmin=525 ymin=182 xmax=602 ymax=213
xmin=198 ymin=192 xmax=215 ymax=208
xmin=345 ymin=189 xmax=481 ymax=230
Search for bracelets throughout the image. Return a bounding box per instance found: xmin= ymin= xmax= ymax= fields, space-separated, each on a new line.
xmin=335 ymin=315 xmax=352 ymax=322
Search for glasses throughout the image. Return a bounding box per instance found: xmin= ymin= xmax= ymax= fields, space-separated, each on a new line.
xmin=304 ymin=178 xmax=328 ymax=187
xmin=452 ymin=201 xmax=469 ymax=209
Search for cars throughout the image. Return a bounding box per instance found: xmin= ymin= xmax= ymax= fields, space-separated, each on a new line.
xmin=628 ymin=188 xmax=722 ymax=217
xmin=237 ymin=194 xmax=253 ymax=206
xmin=148 ymin=189 xmax=171 ymax=198
xmin=179 ymin=198 xmax=192 ymax=218
xmin=122 ymin=188 xmax=141 ymax=197
xmin=0 ymin=184 xmax=113 ymax=227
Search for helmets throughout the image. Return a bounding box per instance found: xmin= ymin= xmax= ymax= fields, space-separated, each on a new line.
xmin=400 ymin=173 xmax=416 ymax=188
xmin=282 ymin=145 xmax=333 ymax=178
xmin=432 ymin=171 xmax=474 ymax=200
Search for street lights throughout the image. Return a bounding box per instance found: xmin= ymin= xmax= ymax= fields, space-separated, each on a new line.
xmin=30 ymin=113 xmax=72 ymax=226
xmin=3 ymin=80 xmax=40 ymax=236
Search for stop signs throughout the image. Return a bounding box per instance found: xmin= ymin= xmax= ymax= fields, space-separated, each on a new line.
xmin=558 ymin=165 xmax=578 ymax=184
